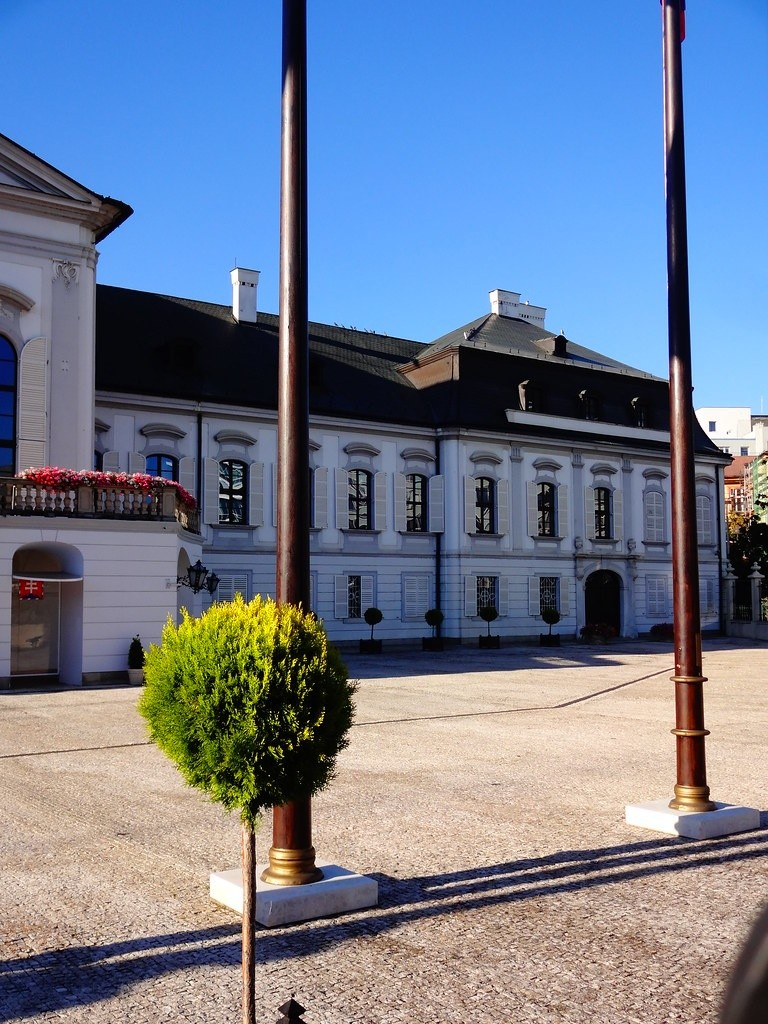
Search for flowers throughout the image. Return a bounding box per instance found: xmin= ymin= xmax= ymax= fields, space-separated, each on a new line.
xmin=650 ymin=623 xmax=672 ymax=640
xmin=580 ymin=623 xmax=618 ymax=640
xmin=15 ymin=466 xmax=198 ymax=510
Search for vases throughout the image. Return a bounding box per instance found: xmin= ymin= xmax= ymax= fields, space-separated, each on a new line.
xmin=656 ymin=633 xmax=664 ymax=641
xmin=592 ymin=634 xmax=608 ymax=645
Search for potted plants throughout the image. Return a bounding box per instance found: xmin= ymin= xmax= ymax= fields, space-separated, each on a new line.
xmin=127 ymin=634 xmax=145 ymax=686
xmin=422 ymin=609 xmax=445 ymax=652
xmin=478 ymin=606 xmax=500 ymax=649
xmin=359 ymin=607 xmax=384 ymax=654
xmin=539 ymin=607 xmax=560 ymax=647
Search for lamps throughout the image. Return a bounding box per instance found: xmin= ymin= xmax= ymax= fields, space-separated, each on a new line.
xmin=200 ymin=572 xmax=221 ymax=596
xmin=177 ymin=560 xmax=210 ymax=595
